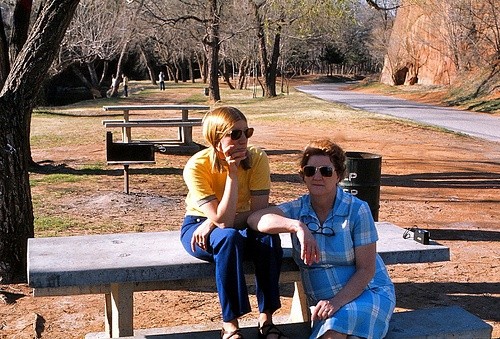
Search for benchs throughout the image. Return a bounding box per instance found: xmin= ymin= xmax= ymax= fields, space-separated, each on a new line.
xmin=105 ymin=122 xmax=203 ymax=143
xmin=84 ymin=304 xmax=493 ymax=339
xmin=102 ymin=118 xmax=202 ymax=143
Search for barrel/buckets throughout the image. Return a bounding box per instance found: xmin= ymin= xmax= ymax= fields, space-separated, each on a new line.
xmin=339 ymin=152 xmax=382 ymax=222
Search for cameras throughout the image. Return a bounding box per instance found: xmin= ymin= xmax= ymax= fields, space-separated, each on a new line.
xmin=414 ymin=229 xmax=430 ymax=245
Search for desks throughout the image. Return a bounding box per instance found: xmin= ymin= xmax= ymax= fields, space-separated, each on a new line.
xmin=26 ymin=221 xmax=493 ymax=338
xmin=103 ymin=105 xmax=210 ymax=143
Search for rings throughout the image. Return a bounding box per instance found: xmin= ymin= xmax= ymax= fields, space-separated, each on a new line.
xmin=198 ymin=242 xmax=203 ymax=244
xmin=325 ymin=309 xmax=328 ymax=312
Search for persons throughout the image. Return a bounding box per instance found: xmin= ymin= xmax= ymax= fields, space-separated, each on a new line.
xmin=122 ymin=73 xmax=129 ymax=97
xmin=180 ymin=107 xmax=284 ymax=339
xmin=159 ymin=71 xmax=165 ymax=90
xmin=247 ymin=139 xmax=396 ymax=339
xmin=111 ymin=74 xmax=119 ymax=97
